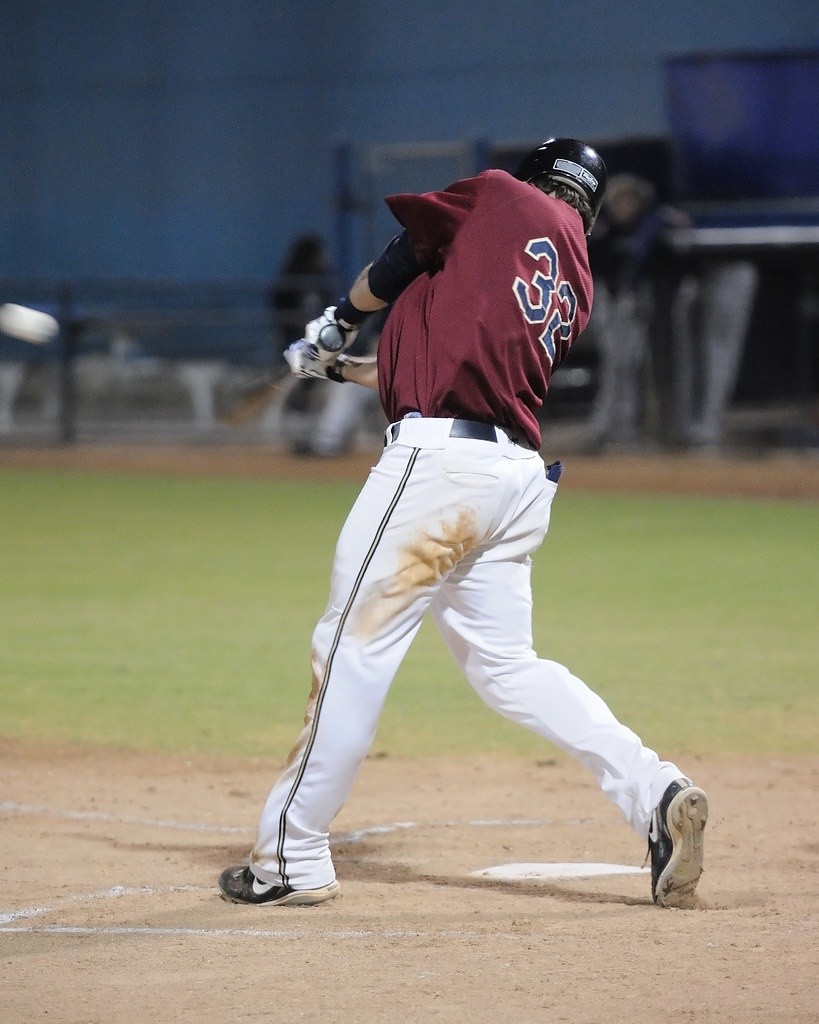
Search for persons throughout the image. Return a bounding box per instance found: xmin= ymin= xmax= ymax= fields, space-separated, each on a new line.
xmin=216 ymin=137 xmax=711 ymax=912
xmin=262 ymin=141 xmax=704 ymax=454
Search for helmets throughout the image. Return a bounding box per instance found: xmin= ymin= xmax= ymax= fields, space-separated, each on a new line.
xmin=517 ymin=137 xmax=607 ymax=221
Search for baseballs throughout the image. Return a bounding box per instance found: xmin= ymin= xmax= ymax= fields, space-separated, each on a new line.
xmin=1 ymin=301 xmax=59 ymax=344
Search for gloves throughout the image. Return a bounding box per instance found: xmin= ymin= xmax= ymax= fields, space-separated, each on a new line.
xmin=283 ymin=336 xmax=353 ymax=383
xmin=304 ymin=306 xmax=363 ymax=363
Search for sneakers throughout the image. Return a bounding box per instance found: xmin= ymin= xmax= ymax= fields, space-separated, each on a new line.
xmin=641 ymin=777 xmax=709 ymax=907
xmin=218 ymin=866 xmax=341 ymax=907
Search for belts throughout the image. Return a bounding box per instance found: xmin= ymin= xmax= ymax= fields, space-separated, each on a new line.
xmin=382 ymin=418 xmax=498 ymax=443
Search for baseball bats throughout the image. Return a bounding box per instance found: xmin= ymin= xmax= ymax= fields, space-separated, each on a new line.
xmin=230 ymin=324 xmax=344 ymax=425
